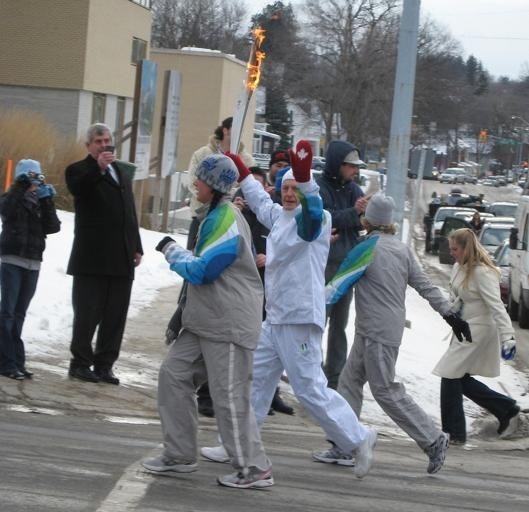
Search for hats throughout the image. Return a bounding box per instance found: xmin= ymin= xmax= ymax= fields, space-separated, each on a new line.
xmin=365 ymin=194 xmax=395 ymax=227
xmin=281 ymin=167 xmax=312 ymax=183
xmin=15 ymin=159 xmax=41 ymax=184
xmin=195 ymin=154 xmax=240 ymax=196
xmin=343 ymin=149 xmax=368 ymax=166
xmin=270 ymin=151 xmax=290 ymax=167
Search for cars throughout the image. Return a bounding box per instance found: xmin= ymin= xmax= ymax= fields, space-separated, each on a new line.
xmin=428 ymin=189 xmax=515 ymax=247
xmin=311 ymin=153 xmax=391 ymax=190
xmin=493 ymin=242 xmax=515 ymax=293
xmin=438 ymin=157 xmax=527 ymax=188
xmin=477 ymin=224 xmax=516 ymax=262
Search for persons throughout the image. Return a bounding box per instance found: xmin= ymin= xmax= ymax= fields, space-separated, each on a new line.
xmin=468 ymin=212 xmax=485 ymax=229
xmin=142 ymin=117 xmax=472 ymax=490
xmin=0 ymin=158 xmax=61 ymax=380
xmin=517 ymin=168 xmax=529 ymax=195
xmin=431 ymin=228 xmax=520 ymax=446
xmin=64 ymin=123 xmax=144 ymax=386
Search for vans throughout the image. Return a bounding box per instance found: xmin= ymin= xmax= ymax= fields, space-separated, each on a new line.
xmin=505 ymin=194 xmax=528 ymax=328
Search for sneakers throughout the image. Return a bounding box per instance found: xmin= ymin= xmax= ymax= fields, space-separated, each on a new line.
xmin=7 ymin=369 xmax=34 ymax=380
xmin=497 ymin=406 xmax=520 ymax=435
xmin=142 ymin=446 xmax=274 ymax=489
xmin=424 ymin=433 xmax=450 ymax=474
xmin=273 ymin=400 xmax=293 ymax=413
xmin=312 ymin=446 xmax=355 ymax=466
xmin=350 ymin=425 xmax=377 ymax=479
xmin=198 ymin=401 xmax=214 ymax=417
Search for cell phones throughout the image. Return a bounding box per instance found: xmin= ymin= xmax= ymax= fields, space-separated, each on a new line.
xmin=104 ymin=145 xmax=115 ymax=154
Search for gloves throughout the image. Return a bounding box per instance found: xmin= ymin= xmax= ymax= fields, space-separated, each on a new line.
xmin=502 ymin=346 xmax=515 ymax=360
xmin=443 ymin=313 xmax=472 ymax=342
xmin=224 ymin=151 xmax=252 ymax=183
xmin=156 ymin=236 xmax=176 ymax=254
xmin=290 ymin=140 xmax=312 ymax=182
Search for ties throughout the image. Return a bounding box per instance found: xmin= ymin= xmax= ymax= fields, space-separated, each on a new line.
xmin=105 ymin=167 xmax=117 ymax=184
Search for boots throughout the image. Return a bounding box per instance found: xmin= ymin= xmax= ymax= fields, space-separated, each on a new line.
xmin=94 ymin=366 xmax=120 ymax=385
xmin=70 ymin=359 xmax=100 ymax=382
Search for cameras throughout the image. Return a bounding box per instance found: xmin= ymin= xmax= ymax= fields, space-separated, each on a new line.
xmin=32 ymin=173 xmax=45 ymax=181
